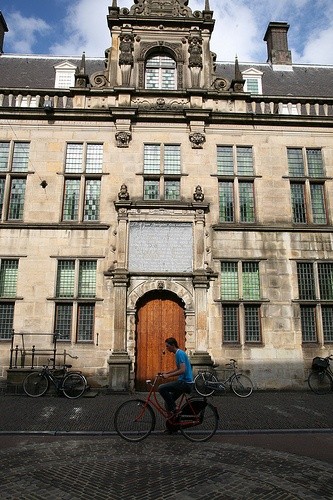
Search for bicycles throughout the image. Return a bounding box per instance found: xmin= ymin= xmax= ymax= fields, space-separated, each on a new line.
xmin=113 ymin=371 xmax=221 ymax=444
xmin=192 ymin=359 xmax=253 ymax=398
xmin=308 ymin=355 xmax=332 ymax=394
xmin=22 ymin=357 xmax=87 ymax=397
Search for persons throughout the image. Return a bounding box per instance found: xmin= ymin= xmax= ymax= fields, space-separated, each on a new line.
xmin=158 ymin=338 xmax=193 ymax=435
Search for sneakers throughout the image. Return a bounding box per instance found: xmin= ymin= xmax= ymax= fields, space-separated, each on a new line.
xmin=165 ymin=407 xmax=182 ymax=421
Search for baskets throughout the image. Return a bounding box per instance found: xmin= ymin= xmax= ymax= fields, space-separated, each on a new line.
xmin=312 ymin=356 xmax=327 ymax=370
